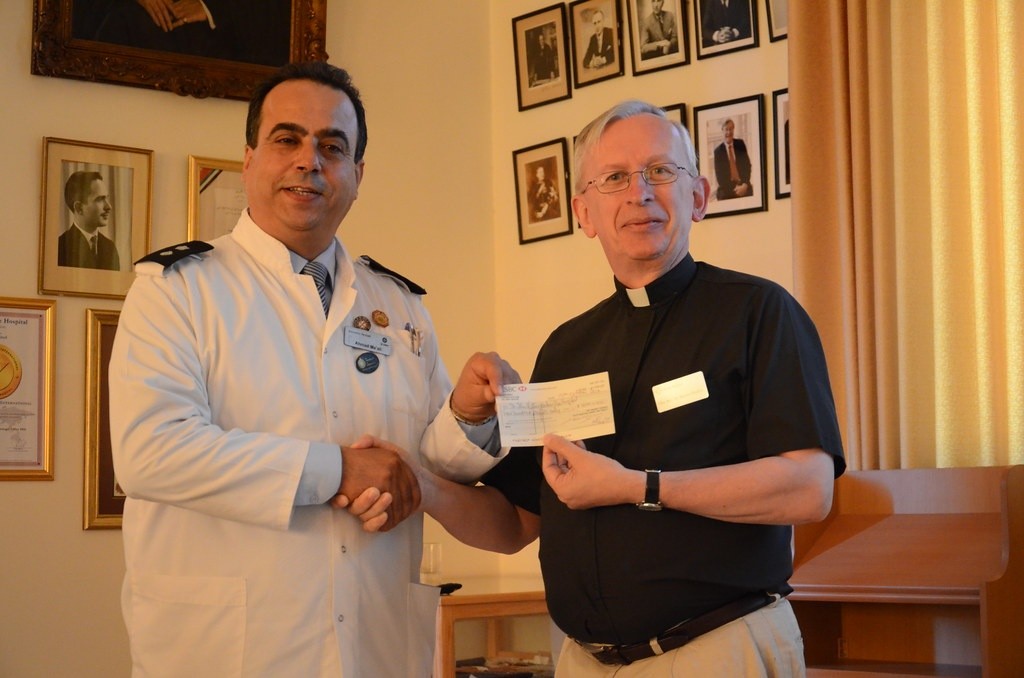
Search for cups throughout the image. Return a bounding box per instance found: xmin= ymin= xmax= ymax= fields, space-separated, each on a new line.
xmin=421 ymin=543 xmax=442 ymax=585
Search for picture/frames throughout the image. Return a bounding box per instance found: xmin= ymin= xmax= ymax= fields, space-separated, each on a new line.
xmin=188 ymin=155 xmax=243 ymax=250
xmin=659 ymin=101 xmax=688 ymax=131
xmin=692 ymin=93 xmax=770 ymax=218
xmin=625 ymin=0 xmax=691 ymax=78
xmin=771 ymin=88 xmax=792 ymax=200
xmin=693 ymin=1 xmax=759 ymax=61
xmin=511 ymin=136 xmax=574 ymax=246
xmin=32 ymin=0 xmax=327 ymax=99
xmin=83 ymin=306 xmax=130 ymax=532
xmin=568 ymin=0 xmax=624 ymax=90
xmin=0 ymin=294 xmax=55 ymax=483
xmin=512 ymin=2 xmax=573 ymax=111
xmin=37 ymin=135 xmax=154 ymax=301
xmin=766 ymin=0 xmax=788 ymax=42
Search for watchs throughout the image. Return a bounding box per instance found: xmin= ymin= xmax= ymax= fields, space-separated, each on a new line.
xmin=635 ymin=469 xmax=662 ymax=512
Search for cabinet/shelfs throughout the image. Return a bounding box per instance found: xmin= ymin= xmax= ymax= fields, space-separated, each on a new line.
xmin=420 ymin=578 xmax=560 ymax=678
xmin=786 ymin=461 xmax=1024 ymax=678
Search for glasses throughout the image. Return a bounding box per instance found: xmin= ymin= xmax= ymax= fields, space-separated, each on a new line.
xmin=577 ymin=163 xmax=696 ymax=193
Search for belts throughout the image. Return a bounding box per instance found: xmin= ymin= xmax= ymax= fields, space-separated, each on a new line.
xmin=566 ymin=583 xmax=795 ymax=666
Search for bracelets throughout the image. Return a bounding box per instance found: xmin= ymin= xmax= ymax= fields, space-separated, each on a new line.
xmin=451 ymin=402 xmax=498 ymax=424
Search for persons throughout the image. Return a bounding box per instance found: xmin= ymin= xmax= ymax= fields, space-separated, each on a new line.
xmin=108 ymin=61 xmax=523 ymax=678
xmin=583 ymin=11 xmax=616 ymax=70
xmin=703 ymin=0 xmax=749 ymax=46
xmin=531 ymin=34 xmax=555 ymax=83
xmin=56 ymin=171 xmax=120 ymax=271
xmin=527 ymin=164 xmax=559 ymax=223
xmin=714 ymin=120 xmax=753 ymax=201
xmin=71 ymin=0 xmax=290 ymax=67
xmin=640 ymin=0 xmax=679 ymax=60
xmin=330 ymin=100 xmax=846 ymax=678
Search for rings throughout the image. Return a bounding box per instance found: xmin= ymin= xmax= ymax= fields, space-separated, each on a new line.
xmin=182 ymin=17 xmax=188 ymax=24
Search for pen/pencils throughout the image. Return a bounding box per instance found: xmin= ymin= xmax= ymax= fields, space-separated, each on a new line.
xmin=414 ymin=327 xmax=424 ymax=356
xmin=405 ymin=322 xmax=416 ymax=353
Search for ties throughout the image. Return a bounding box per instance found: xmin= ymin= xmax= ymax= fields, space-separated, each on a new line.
xmin=90 ymin=236 xmax=98 ymax=257
xmin=302 ymin=261 xmax=329 ymax=318
xmin=659 ymin=16 xmax=663 ymax=32
xmin=728 ymin=144 xmax=739 ymax=182
